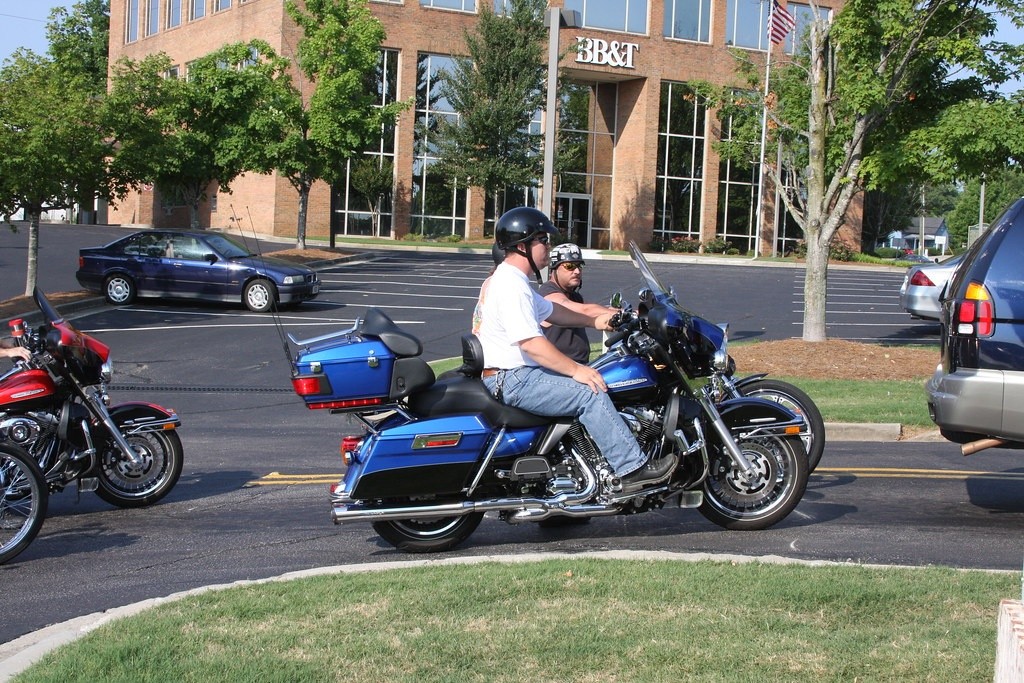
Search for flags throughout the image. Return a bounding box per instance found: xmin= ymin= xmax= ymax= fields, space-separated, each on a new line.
xmin=767 ymin=0 xmax=795 ymax=46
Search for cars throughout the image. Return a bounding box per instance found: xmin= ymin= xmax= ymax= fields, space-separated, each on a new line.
xmin=897 ymin=250 xmax=967 ymax=321
xmin=75 ymin=229 xmax=322 ymax=313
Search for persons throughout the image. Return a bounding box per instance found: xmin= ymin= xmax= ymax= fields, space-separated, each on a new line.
xmin=536 ymin=244 xmax=638 ymax=366
xmin=472 ymin=207 xmax=679 ymax=526
xmin=0 ymin=340 xmax=33 ymax=364
xmin=489 ymin=242 xmax=505 ymax=275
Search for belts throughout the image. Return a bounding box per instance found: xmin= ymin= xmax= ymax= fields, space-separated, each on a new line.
xmin=482 ymin=367 xmax=506 ymax=377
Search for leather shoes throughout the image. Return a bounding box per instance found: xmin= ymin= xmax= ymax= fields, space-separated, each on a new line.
xmin=620 ymin=453 xmax=679 ymax=493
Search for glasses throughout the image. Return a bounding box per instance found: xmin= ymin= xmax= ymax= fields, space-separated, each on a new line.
xmin=559 ymin=262 xmax=584 ymax=271
xmin=532 ymin=233 xmax=551 ymax=244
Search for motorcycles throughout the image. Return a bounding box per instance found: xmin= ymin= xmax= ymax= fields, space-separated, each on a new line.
xmin=283 ymin=238 xmax=818 ymax=552
xmin=362 ymin=240 xmax=827 ymax=487
xmin=0 ymin=285 xmax=183 ymax=566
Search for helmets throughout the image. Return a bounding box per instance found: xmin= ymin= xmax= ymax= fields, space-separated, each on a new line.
xmin=492 ymin=241 xmax=506 ymax=265
xmin=494 ymin=206 xmax=560 ymax=250
xmin=548 ymin=243 xmax=585 ymax=268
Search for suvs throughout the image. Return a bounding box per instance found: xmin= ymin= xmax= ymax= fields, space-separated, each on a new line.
xmin=925 ymin=197 xmax=1024 ymax=455
